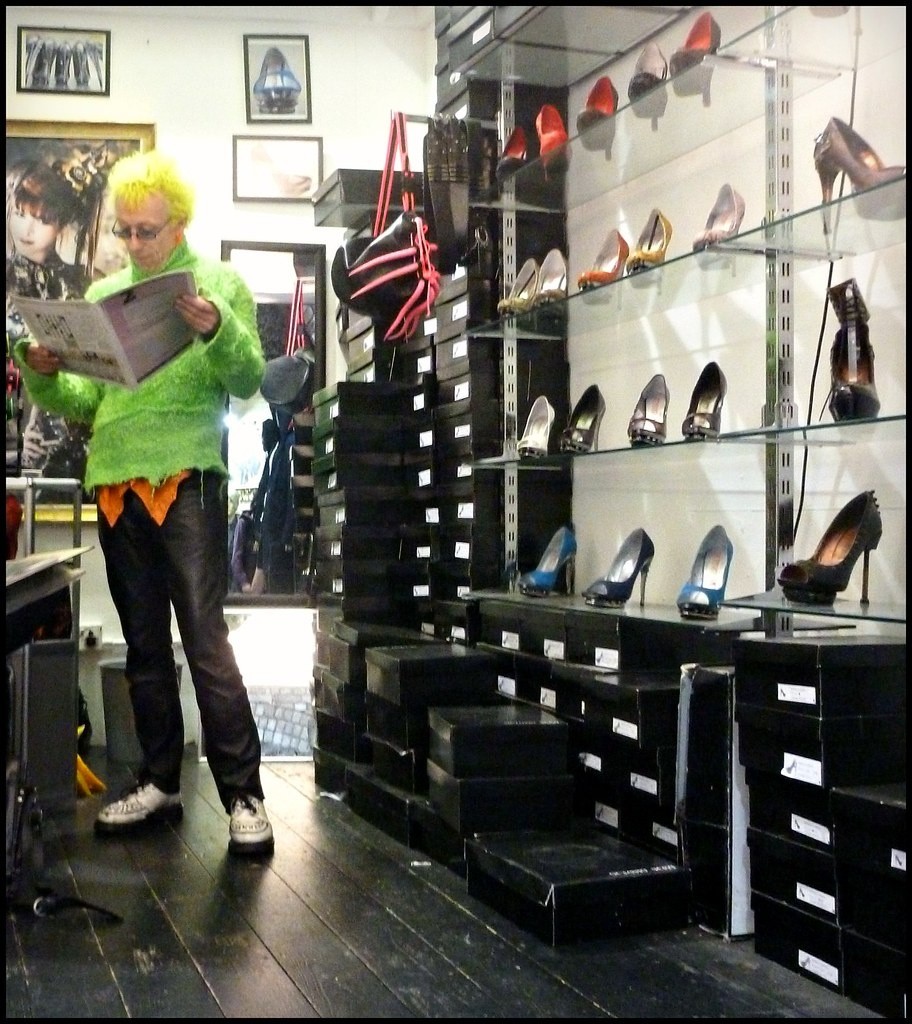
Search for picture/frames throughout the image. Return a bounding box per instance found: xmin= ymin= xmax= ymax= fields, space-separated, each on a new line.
xmin=230 ymin=132 xmax=325 ymax=201
xmin=15 ymin=24 xmax=112 ymax=97
xmin=6 ymin=118 xmax=161 ymax=525
xmin=241 ymin=31 xmax=314 ymax=127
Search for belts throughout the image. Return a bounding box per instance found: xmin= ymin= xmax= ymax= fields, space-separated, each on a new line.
xmin=423 ymin=113 xmax=489 ymax=277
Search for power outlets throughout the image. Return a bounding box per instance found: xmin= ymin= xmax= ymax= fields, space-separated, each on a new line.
xmin=77 ymin=620 xmax=103 ymax=655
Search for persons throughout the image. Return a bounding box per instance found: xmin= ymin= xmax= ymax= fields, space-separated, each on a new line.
xmin=14 ymin=150 xmax=275 ymax=857
xmin=252 ymin=419 xmax=293 ymax=593
xmin=7 ymin=142 xmax=116 ymax=461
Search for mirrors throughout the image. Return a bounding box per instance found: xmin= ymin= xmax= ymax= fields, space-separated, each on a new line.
xmin=216 ymin=237 xmax=330 ymax=610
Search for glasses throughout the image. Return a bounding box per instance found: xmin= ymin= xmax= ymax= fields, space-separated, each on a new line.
xmin=112 ymin=217 xmax=172 ymax=241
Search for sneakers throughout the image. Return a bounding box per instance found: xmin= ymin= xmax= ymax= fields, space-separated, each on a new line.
xmin=229 ymin=793 xmax=272 ymax=844
xmin=97 ymin=783 xmax=183 ymax=824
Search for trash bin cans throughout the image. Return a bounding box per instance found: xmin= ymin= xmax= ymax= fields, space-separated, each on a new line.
xmin=99 ymin=660 xmax=182 ymax=765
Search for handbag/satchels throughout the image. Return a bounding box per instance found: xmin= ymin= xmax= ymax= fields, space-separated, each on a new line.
xmin=330 ymin=112 xmax=441 ymax=341
xmin=260 ymin=281 xmax=313 ymax=410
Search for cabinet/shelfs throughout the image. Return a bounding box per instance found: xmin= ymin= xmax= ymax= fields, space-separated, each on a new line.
xmin=459 ymin=0 xmax=912 ymax=635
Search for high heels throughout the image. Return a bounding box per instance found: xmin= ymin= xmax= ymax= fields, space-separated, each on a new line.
xmin=491 ymin=13 xmax=906 ymax=626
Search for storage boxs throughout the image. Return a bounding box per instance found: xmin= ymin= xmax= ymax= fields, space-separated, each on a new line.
xmin=287 ymin=413 xmax=312 ymax=445
xmin=293 ymin=508 xmax=313 ymax=536
xmin=289 ymin=475 xmax=313 ymax=508
xmin=308 ymin=6 xmax=911 ymax=1019
xmin=288 ymin=444 xmax=313 ymax=476
xmin=292 ymin=535 xmax=310 ymax=562
